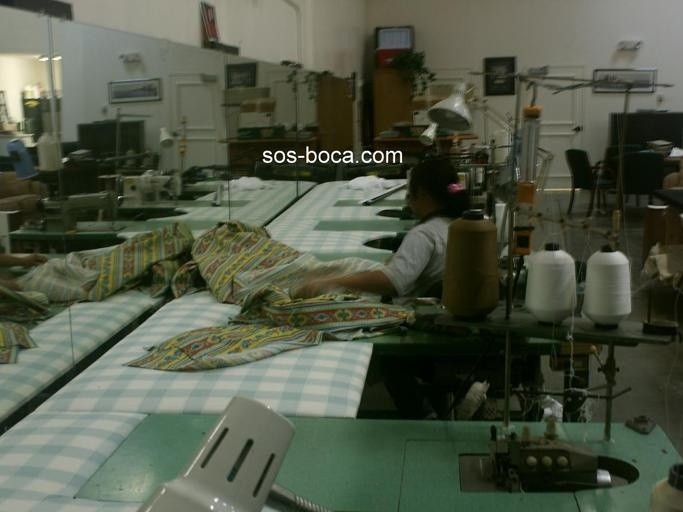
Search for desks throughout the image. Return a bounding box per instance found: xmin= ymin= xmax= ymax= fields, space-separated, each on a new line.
xmin=660 ymin=156 xmax=683 ymax=172
xmin=0 ymin=179 xmax=317 ymax=432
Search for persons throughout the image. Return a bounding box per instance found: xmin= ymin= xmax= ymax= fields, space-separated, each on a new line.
xmin=290 ymin=156 xmax=467 ymax=419
xmin=0 ymin=252 xmax=47 ymax=267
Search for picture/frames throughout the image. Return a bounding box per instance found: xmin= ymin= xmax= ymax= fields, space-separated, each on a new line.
xmin=108 ymin=77 xmax=161 ymax=103
xmin=592 ymin=67 xmax=658 ymax=93
xmin=225 ymin=62 xmax=256 ymax=88
xmin=483 ymin=56 xmax=517 ymax=97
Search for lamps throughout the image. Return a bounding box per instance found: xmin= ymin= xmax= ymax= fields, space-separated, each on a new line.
xmin=159 ymin=127 xmax=175 ymax=147
xmin=429 ymin=83 xmax=473 ymax=131
xmin=420 ymin=121 xmax=436 ymax=144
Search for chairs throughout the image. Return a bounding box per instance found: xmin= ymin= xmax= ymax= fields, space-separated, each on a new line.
xmin=598 ymin=145 xmax=643 ymax=208
xmin=616 ymin=154 xmax=664 ymax=213
xmin=564 ymin=148 xmax=593 ymax=213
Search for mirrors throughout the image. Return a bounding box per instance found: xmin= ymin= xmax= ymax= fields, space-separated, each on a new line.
xmin=0 ymin=6 xmax=353 ymax=441
